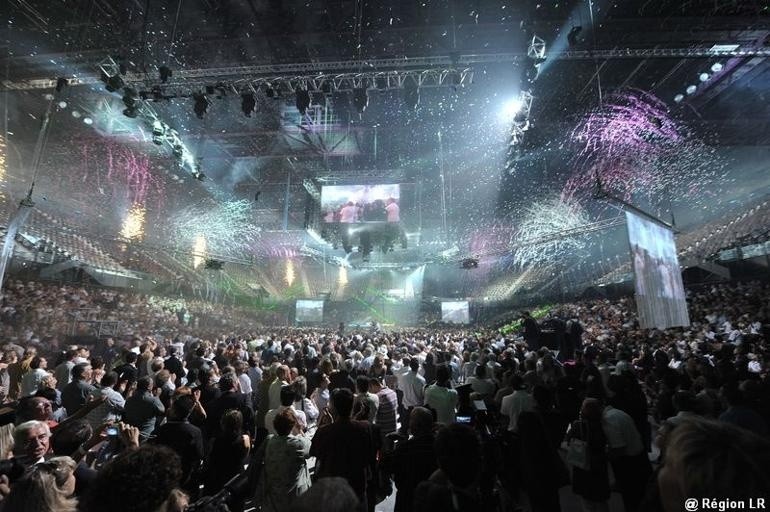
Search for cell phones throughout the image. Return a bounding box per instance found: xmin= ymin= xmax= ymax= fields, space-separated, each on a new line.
xmin=105 ymin=424 xmax=121 ymax=437
xmin=457 ymin=413 xmax=474 ymax=426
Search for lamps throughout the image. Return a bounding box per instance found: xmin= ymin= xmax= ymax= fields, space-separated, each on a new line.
xmin=41 ymin=0 xmax=739 ymax=181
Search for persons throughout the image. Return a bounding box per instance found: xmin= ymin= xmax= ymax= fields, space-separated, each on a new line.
xmin=323 ymin=197 xmax=400 ymax=223
xmin=2 ymin=180 xmax=186 ymax=263
xmin=590 ymin=194 xmax=770 ymax=284
xmin=0 ymin=262 xmax=770 ymax=511
